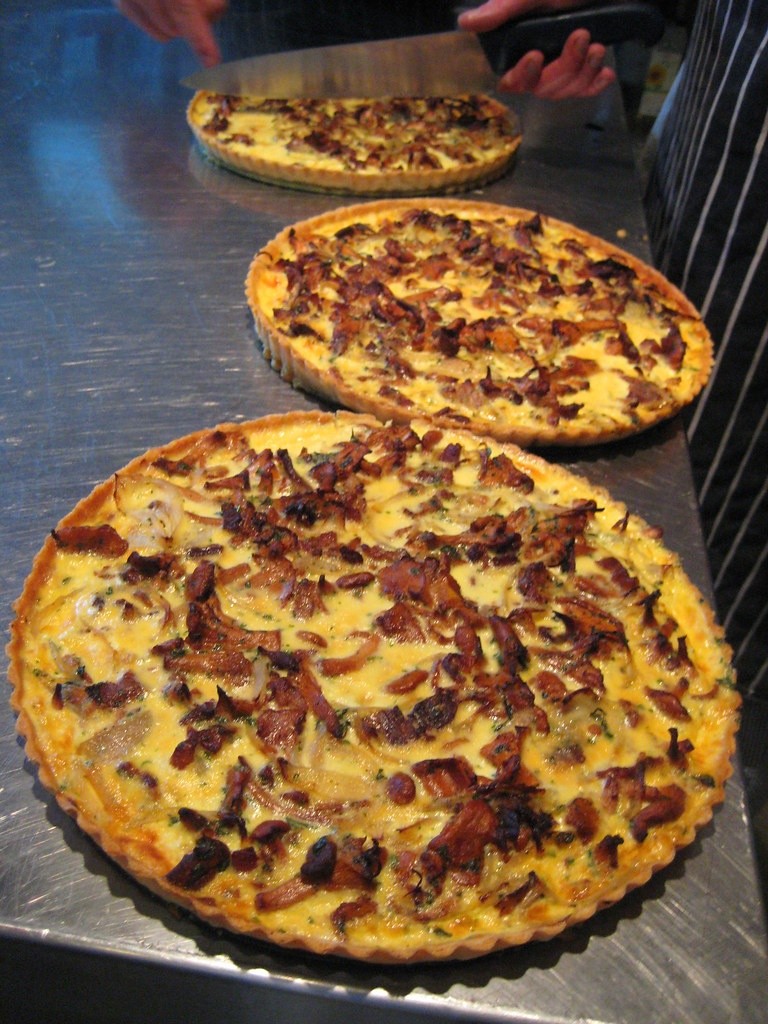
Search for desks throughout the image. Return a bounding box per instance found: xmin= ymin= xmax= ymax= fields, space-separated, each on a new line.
xmin=0 ymin=0 xmax=768 ymax=1024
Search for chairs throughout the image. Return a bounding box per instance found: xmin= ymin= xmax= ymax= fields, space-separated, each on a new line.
xmin=638 ymin=0 xmax=768 ymax=760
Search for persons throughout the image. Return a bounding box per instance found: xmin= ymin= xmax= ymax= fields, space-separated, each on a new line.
xmin=458 ymin=0 xmax=768 ymax=753
xmin=114 ymin=0 xmax=661 ymax=130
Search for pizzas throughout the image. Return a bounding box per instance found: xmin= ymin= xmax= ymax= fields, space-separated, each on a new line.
xmin=184 ymin=74 xmax=522 ymax=191
xmin=242 ymin=194 xmax=716 ymax=444
xmin=6 ymin=408 xmax=743 ymax=959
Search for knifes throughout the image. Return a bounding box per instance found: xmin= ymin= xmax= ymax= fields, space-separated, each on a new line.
xmin=179 ymin=0 xmax=688 ymax=100
